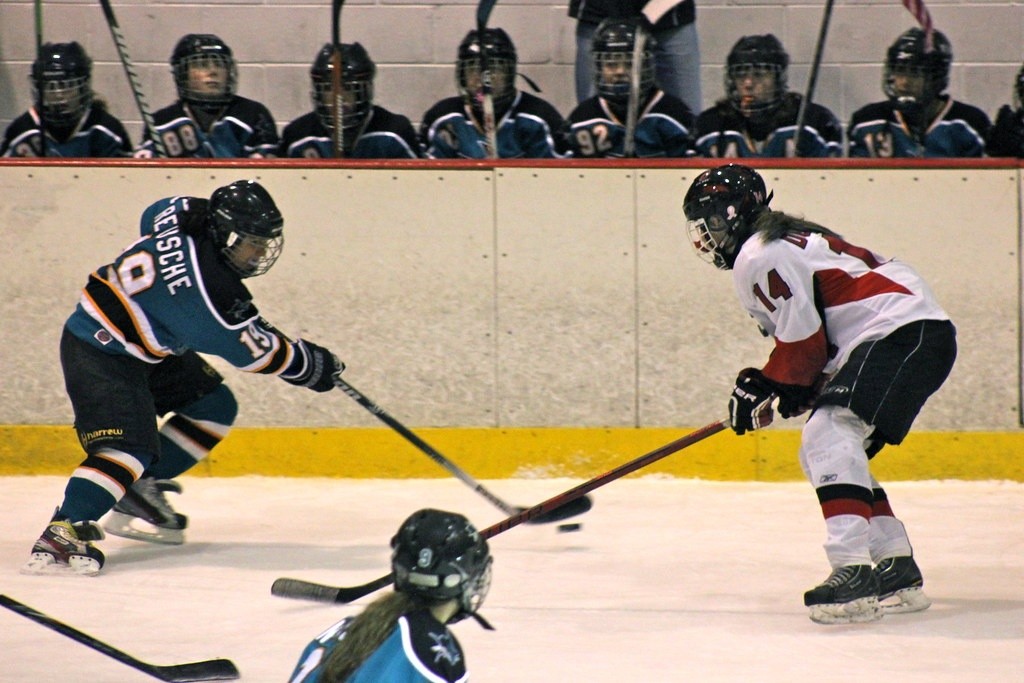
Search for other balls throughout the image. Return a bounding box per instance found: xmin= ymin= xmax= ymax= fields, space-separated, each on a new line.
xmin=559 ymin=523 xmax=580 ymax=532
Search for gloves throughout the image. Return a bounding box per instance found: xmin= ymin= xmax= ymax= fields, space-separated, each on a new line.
xmin=278 ymin=338 xmax=345 ymax=392
xmin=727 ymin=368 xmax=783 ymax=435
xmin=777 ymin=372 xmax=833 ymax=418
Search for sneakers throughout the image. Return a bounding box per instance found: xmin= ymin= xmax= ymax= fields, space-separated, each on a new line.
xmin=104 ymin=477 xmax=188 ymax=543
xmin=22 ymin=506 xmax=105 ymax=576
xmin=871 ymin=554 xmax=931 ymax=614
xmin=804 ymin=564 xmax=883 ymax=624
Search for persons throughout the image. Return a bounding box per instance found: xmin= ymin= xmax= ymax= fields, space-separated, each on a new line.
xmin=289 ymin=510 xmax=494 ymax=683
xmin=20 ymin=179 xmax=346 ymax=576
xmin=685 ymin=161 xmax=957 ymax=622
xmin=848 ymin=27 xmax=990 ymax=158
xmin=984 ymin=64 xmax=1024 ymax=159
xmin=0 ymin=41 xmax=134 ymax=159
xmin=282 ymin=41 xmax=421 ymax=159
xmin=568 ymin=0 xmax=701 ymax=113
xmin=419 ymin=28 xmax=581 ymax=158
xmin=135 ymin=34 xmax=280 ymax=160
xmin=692 ymin=35 xmax=844 ymax=158
xmin=564 ymin=16 xmax=696 ymax=160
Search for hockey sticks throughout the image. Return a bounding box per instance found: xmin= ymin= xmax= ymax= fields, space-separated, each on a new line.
xmin=790 ymin=0 xmax=836 ymax=160
xmin=902 ymin=0 xmax=934 ymax=159
xmin=270 ymin=417 xmax=735 ymax=606
xmin=257 ymin=316 xmax=594 ymax=524
xmin=32 ymin=1 xmax=48 ymax=158
xmin=623 ymin=0 xmax=684 ymax=159
xmin=476 ymin=0 xmax=498 ymax=159
xmin=330 ymin=0 xmax=345 ymax=158
xmin=1 ymin=594 xmax=241 ymax=683
xmin=99 ymin=0 xmax=169 ymax=158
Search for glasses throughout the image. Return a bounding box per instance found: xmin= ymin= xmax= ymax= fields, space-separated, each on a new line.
xmin=699 ymin=214 xmax=727 ymax=231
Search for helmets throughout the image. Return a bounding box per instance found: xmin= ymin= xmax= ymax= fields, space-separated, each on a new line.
xmin=204 ymin=181 xmax=284 ymax=278
xmin=388 ymin=509 xmax=493 ymax=623
xmin=591 ymin=14 xmax=659 ymax=105
xmin=684 ymin=164 xmax=767 ymax=269
xmin=312 ymin=42 xmax=377 ymax=131
xmin=30 ymin=41 xmax=91 ymax=121
xmin=458 ymin=28 xmax=517 ymax=117
xmin=170 ymin=33 xmax=233 ymax=106
xmin=889 ymin=27 xmax=952 ymax=112
xmin=727 ymin=34 xmax=790 ymax=125
